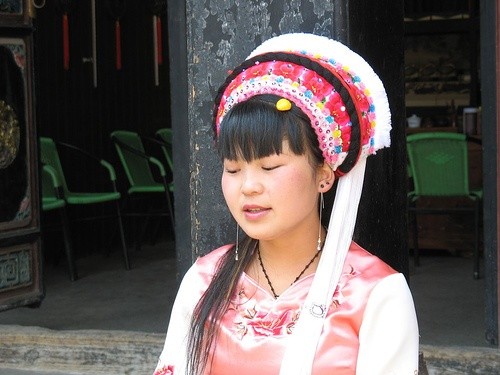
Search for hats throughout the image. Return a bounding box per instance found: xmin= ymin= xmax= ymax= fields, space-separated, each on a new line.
xmin=210 ymin=32 xmax=392 ymax=375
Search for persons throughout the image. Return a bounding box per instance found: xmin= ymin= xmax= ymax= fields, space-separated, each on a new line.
xmin=155 ymin=32 xmax=420 ymax=375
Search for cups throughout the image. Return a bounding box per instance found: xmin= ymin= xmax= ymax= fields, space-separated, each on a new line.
xmin=408 ymin=115 xmax=420 ymax=128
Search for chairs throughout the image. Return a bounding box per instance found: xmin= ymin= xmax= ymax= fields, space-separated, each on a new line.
xmin=407 ymin=131 xmax=483 ymax=281
xmin=38 ymin=127 xmax=176 ymax=283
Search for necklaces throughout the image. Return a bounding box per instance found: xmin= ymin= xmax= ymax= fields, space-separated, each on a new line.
xmin=257 ymin=226 xmax=327 ymax=300
